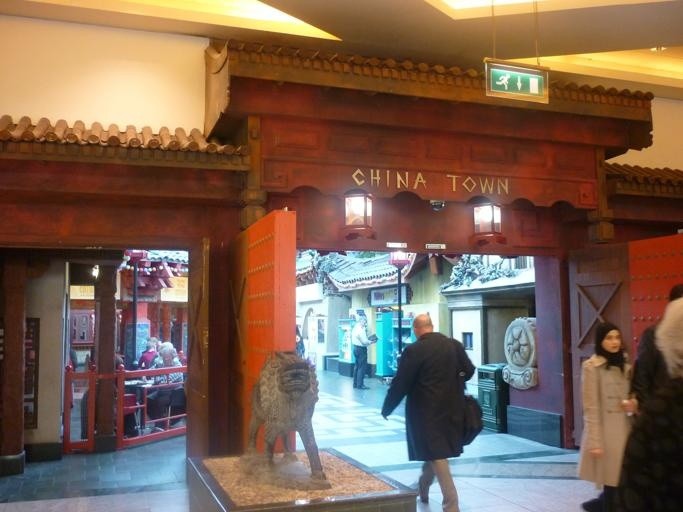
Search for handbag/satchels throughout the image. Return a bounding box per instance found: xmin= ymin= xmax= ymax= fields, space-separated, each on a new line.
xmin=463 ymin=394 xmax=484 ymax=445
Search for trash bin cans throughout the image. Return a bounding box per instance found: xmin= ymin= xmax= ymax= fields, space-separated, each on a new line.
xmin=478 ymin=362 xmax=510 ymax=434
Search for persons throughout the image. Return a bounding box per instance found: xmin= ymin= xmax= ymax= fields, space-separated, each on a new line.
xmin=351 ymin=315 xmax=377 ymax=390
xmin=626 ymin=283 xmax=682 ymax=407
xmin=380 ymin=313 xmax=477 ymax=512
xmin=112 ymin=335 xmax=187 ymax=439
xmin=294 ymin=323 xmax=308 ymax=358
xmin=615 ymin=292 xmax=682 ymax=510
xmin=574 ymin=321 xmax=641 ymax=510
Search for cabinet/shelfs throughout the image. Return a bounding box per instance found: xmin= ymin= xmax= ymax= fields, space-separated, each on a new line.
xmin=391 ymin=318 xmax=417 ymax=371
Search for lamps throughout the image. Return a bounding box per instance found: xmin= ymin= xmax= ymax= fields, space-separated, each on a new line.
xmin=469 ymin=203 xmax=507 ymax=248
xmin=340 ymin=192 xmax=377 ymax=243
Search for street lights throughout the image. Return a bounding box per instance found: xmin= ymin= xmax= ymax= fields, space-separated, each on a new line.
xmin=388 ymin=251 xmax=411 ymax=369
xmin=125 ymin=250 xmax=151 ymax=414
